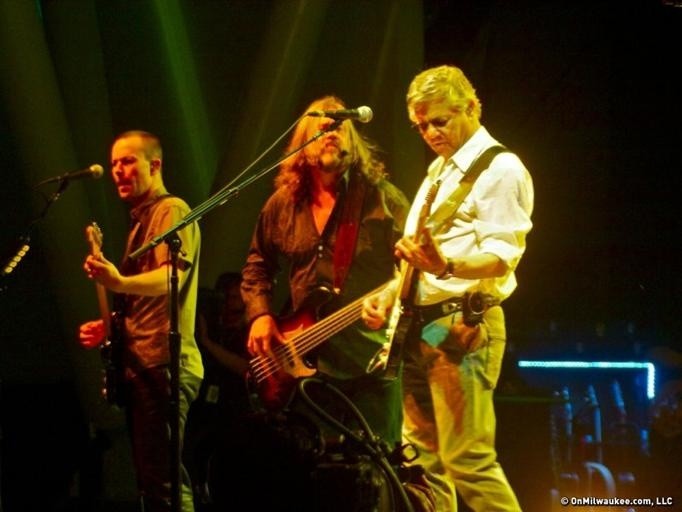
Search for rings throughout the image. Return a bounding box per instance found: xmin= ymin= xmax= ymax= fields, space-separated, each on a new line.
xmin=364 ymin=320 xmax=368 ymax=329
xmin=86 ymin=268 xmax=92 ymax=274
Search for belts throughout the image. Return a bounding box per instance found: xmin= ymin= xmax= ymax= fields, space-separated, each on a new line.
xmin=414 ymin=292 xmax=499 ymax=324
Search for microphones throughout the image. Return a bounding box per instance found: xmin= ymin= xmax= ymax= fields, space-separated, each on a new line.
xmin=42 ymin=163 xmax=105 ymax=185
xmin=308 ymin=104 xmax=374 ymax=123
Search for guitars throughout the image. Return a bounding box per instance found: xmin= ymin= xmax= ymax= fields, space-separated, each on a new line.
xmin=248 ymin=279 xmax=391 ymax=412
xmin=85 ymin=222 xmax=122 ymax=406
xmin=374 ymin=180 xmax=442 ymax=377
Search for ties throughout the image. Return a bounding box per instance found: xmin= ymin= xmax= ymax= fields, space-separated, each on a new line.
xmin=400 ymin=162 xmax=455 ymax=300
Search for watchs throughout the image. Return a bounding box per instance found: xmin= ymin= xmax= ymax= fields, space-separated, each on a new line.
xmin=437 ymin=262 xmax=454 ymax=281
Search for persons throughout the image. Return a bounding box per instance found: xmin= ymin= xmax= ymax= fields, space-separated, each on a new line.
xmin=237 ymin=95 xmax=414 ymax=511
xmin=77 ymin=130 xmax=204 ymax=512
xmin=192 ymin=265 xmax=265 ymax=509
xmin=360 ymin=63 xmax=533 ymax=511
